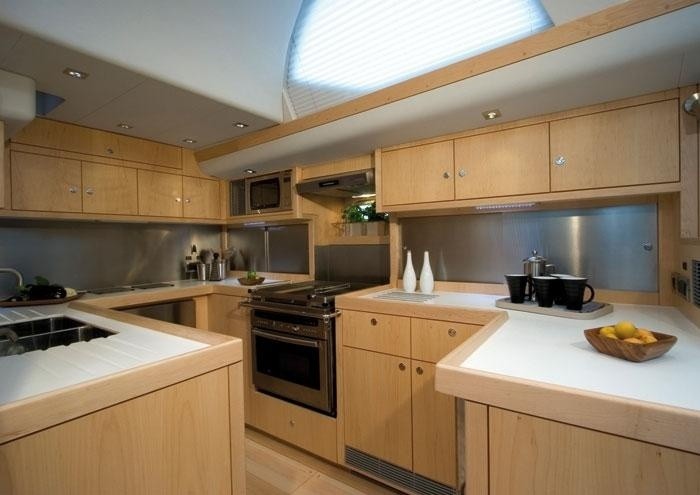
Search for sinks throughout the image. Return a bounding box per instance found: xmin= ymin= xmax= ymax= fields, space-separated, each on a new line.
xmin=0 ymin=316 xmax=90 ymax=343
xmin=1 ymin=325 xmax=118 ymax=357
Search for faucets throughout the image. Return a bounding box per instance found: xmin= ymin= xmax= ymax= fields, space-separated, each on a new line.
xmin=0 ymin=328 xmax=17 ymax=343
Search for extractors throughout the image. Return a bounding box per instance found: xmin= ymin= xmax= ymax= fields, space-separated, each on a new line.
xmin=295 ymin=168 xmax=373 ymax=201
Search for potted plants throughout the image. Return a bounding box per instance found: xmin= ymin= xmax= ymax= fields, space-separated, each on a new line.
xmin=339 ymin=203 xmax=367 ymax=236
xmin=362 ymin=201 xmax=387 ymax=239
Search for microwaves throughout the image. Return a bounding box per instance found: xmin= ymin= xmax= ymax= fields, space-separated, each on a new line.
xmin=244 ymin=169 xmax=293 ymax=215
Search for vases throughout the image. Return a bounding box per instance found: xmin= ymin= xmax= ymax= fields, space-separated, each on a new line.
xmin=402 ymin=249 xmax=417 ymax=291
xmin=420 ymin=251 xmax=435 ymax=293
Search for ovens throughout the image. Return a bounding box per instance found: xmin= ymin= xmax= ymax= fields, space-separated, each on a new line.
xmin=238 ymin=280 xmax=384 ymax=417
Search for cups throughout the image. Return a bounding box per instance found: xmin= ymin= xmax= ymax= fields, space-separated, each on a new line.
xmin=532 ymin=274 xmax=560 ymax=309
xmin=550 ymin=272 xmax=570 ymax=306
xmin=560 ymin=275 xmax=594 ymax=310
xmin=217 ymin=263 xmax=226 ymax=278
xmin=196 ymin=263 xmax=207 ymax=281
xmin=503 ymin=272 xmax=527 ymax=304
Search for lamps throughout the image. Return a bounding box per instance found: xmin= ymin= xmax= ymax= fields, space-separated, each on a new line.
xmin=244 ymin=169 xmax=255 ymax=175
xmin=183 ymin=138 xmax=199 ymax=145
xmin=233 ymin=122 xmax=248 ymax=130
xmin=62 ymin=67 xmax=89 ymax=82
xmin=481 ymin=109 xmax=501 ymax=120
xmin=119 ymin=124 xmax=134 ymax=131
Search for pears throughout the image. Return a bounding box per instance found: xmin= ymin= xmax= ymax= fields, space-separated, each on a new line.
xmin=600 ymin=327 xmax=615 ymax=336
xmin=641 ymin=335 xmax=658 ymax=343
xmin=616 ymin=321 xmax=636 ymax=338
xmin=605 ymin=333 xmax=619 ymax=339
xmin=636 ymin=328 xmax=654 ymax=339
xmin=624 ymin=338 xmax=642 ymax=344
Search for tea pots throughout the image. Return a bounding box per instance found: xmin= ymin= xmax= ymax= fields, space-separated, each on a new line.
xmin=523 ymin=249 xmax=555 ymax=300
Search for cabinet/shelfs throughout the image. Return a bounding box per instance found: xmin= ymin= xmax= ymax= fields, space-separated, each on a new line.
xmin=334 ymin=278 xmax=700 ymax=495
xmin=10 ymin=149 xmax=139 ymax=216
xmin=208 ymin=293 xmax=251 ymax=427
xmin=137 ymin=167 xmax=221 ymax=220
xmin=2 ymin=302 xmax=247 ymax=495
xmin=549 ymin=98 xmax=678 ymax=199
xmin=250 ymin=391 xmax=337 ymax=464
xmin=382 ymin=121 xmax=551 ymax=208
xmin=10 ymin=116 xmax=183 ymax=170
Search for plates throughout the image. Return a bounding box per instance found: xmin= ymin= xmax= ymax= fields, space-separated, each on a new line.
xmin=494 ymin=295 xmax=617 ymax=320
xmin=0 ymin=289 xmax=86 ymax=307
xmin=237 ymin=275 xmax=265 ymax=286
xmin=583 ymin=325 xmax=678 ymax=364
